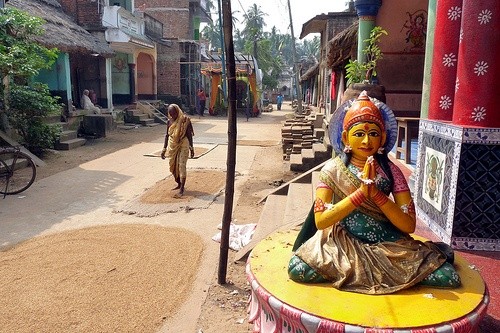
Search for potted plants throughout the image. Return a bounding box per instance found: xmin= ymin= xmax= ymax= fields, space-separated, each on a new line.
xmin=341 ymin=25 xmax=388 ymax=104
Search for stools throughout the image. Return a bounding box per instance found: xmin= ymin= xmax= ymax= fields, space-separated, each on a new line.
xmin=397 ymin=116 xmax=420 ymax=163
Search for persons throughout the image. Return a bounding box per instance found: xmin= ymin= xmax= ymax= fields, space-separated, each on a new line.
xmin=288 ymin=91 xmax=462 ymax=288
xmin=161 ymin=104 xmax=194 ymax=199
xmin=82 ymin=88 xmax=100 ymax=115
xmin=198 ymin=88 xmax=207 ymax=118
xmin=276 ymin=93 xmax=283 ymax=110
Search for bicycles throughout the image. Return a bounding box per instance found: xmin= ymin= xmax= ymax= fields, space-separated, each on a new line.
xmin=0 ymin=145 xmax=37 ymax=199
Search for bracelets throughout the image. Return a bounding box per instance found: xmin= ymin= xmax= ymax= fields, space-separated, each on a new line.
xmin=190 ymin=147 xmax=194 ymax=150
xmin=162 ymin=148 xmax=166 ymax=152
